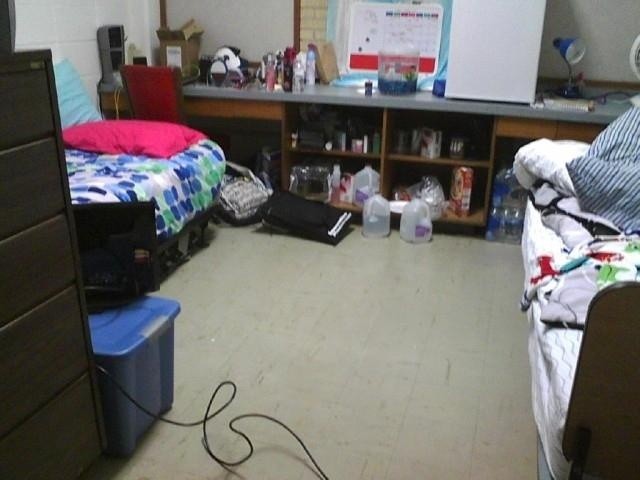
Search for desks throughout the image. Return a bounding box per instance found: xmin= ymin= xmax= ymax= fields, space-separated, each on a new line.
xmin=102 ymin=79 xmax=639 ymax=240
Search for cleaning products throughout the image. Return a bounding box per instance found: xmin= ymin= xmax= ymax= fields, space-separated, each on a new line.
xmin=331 ymin=165 xmax=342 ymax=203
xmin=307 ymin=43 xmax=316 ymax=86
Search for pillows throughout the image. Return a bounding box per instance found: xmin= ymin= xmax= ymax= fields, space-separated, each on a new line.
xmin=62 ymin=119 xmax=210 ymax=160
xmin=567 ymin=105 xmax=640 ymax=235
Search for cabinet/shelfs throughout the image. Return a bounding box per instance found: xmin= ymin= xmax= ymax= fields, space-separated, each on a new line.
xmin=2 ymin=48 xmax=108 ymax=480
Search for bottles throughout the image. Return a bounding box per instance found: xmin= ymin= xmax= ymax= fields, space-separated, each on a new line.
xmin=486 ymin=206 xmax=522 ymax=241
xmin=306 ymin=49 xmax=316 ymax=88
xmin=332 ymin=165 xmax=341 ymax=201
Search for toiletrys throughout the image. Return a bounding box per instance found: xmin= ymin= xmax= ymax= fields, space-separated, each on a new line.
xmin=253 ymin=40 xmax=319 ymax=93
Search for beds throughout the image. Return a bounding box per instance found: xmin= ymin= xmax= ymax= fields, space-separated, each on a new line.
xmin=64 ymin=140 xmax=226 ymax=266
xmin=513 ymin=140 xmax=640 ymax=480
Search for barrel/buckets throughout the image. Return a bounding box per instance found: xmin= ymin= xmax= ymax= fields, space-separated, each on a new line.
xmin=353 ymin=165 xmax=380 ymax=207
xmin=399 ymin=194 xmax=432 ymax=243
xmin=362 ymin=191 xmax=391 ymax=237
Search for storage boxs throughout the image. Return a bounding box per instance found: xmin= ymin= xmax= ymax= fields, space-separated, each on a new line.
xmin=157 ymin=21 xmax=203 ymax=77
xmin=88 ymin=295 xmax=181 ymax=457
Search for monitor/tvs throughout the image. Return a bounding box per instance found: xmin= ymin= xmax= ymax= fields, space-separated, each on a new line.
xmin=72 ymin=199 xmax=162 ymax=317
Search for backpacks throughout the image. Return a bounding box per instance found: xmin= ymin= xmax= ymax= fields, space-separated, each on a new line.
xmin=218 ymin=161 xmax=352 ymax=246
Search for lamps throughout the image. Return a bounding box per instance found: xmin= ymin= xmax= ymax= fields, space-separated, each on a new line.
xmin=553 ymin=37 xmax=587 ymax=100
